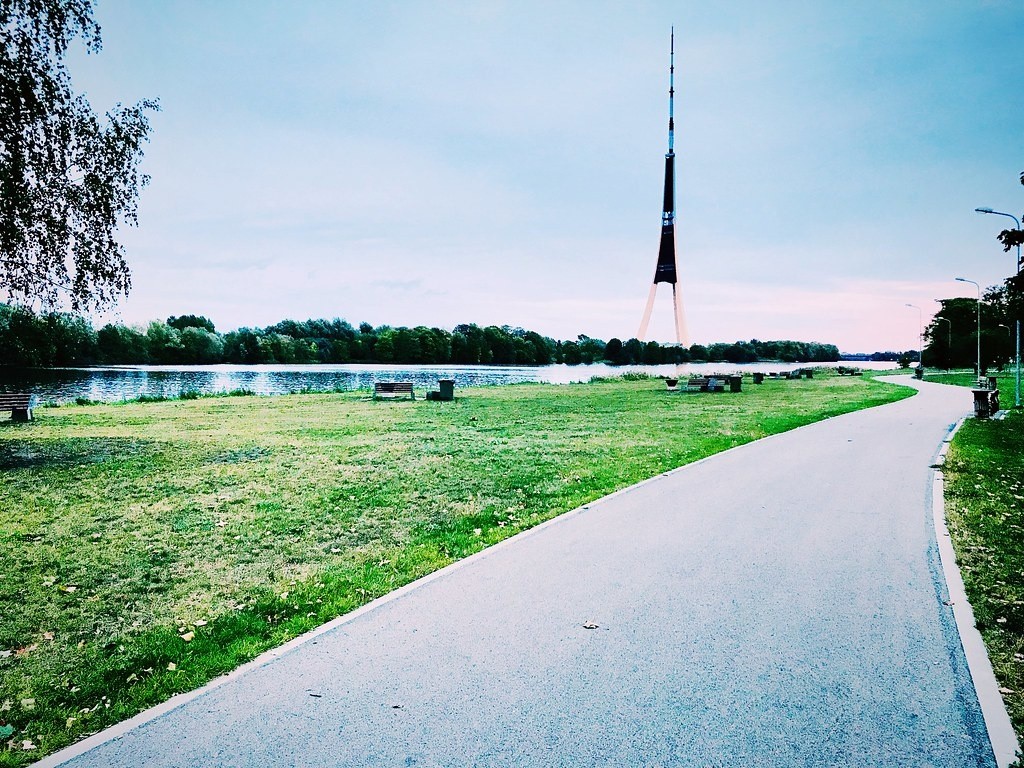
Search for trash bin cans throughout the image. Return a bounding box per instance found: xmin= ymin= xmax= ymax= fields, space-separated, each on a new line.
xmin=917 ymin=370 xmax=923 ymax=380
xmin=754 ymin=373 xmax=763 ymax=384
xmin=438 ymin=379 xmax=455 ymax=399
xmin=972 ymin=389 xmax=999 ymax=418
xmin=987 ymin=376 xmax=998 ymax=390
xmin=729 ymin=376 xmax=741 ymax=393
xmin=806 ymin=370 xmax=813 ymax=378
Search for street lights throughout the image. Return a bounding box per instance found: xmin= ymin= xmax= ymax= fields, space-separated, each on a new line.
xmin=973 ymin=207 xmax=1021 ymax=409
xmin=955 ymin=276 xmax=981 ymax=382
xmin=904 ymin=302 xmax=923 ymax=369
xmin=938 ymin=316 xmax=952 ymax=350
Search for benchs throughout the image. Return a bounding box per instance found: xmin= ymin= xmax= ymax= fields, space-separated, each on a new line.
xmin=0 ymin=394 xmax=33 ymax=421
xmin=373 ymin=383 xmax=415 ymax=400
xmin=686 ymin=379 xmax=725 ymax=393
